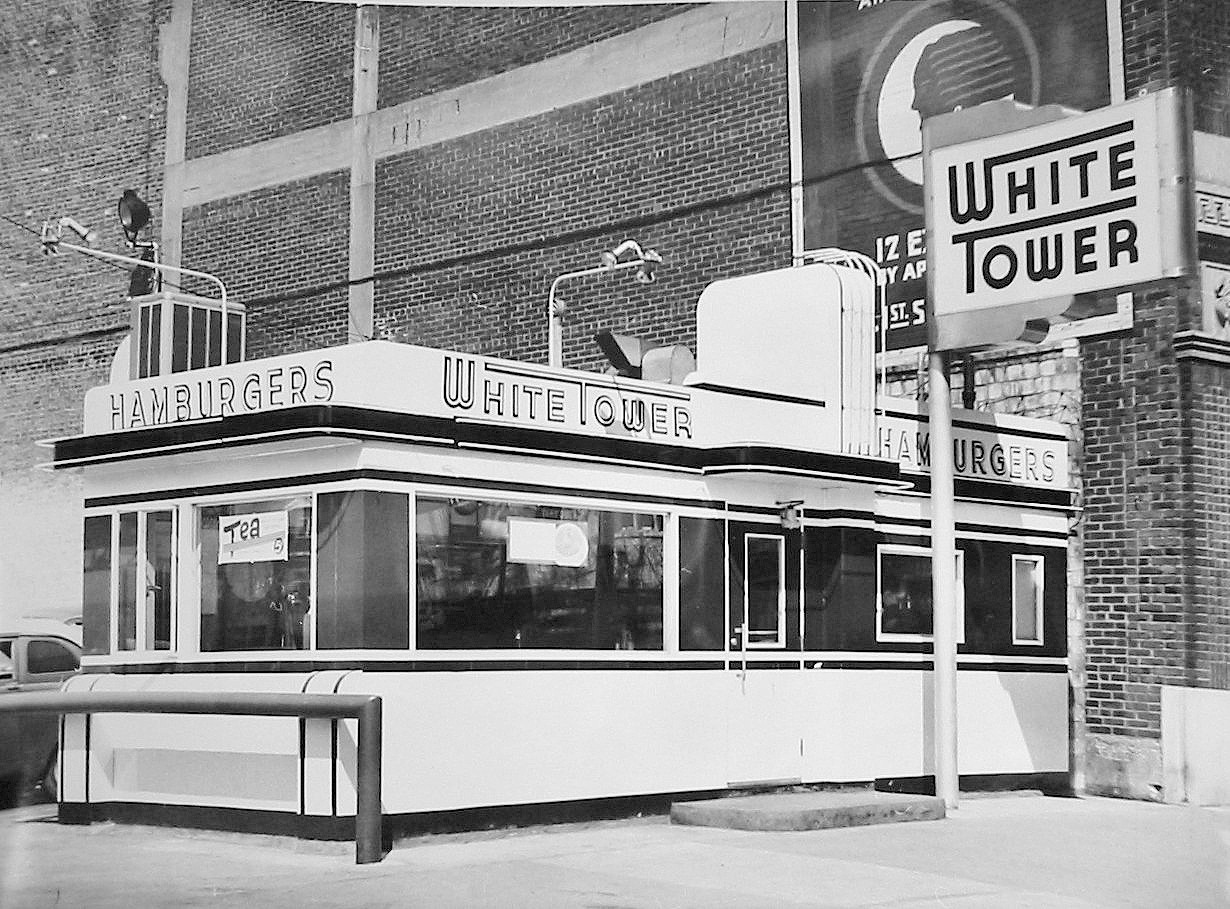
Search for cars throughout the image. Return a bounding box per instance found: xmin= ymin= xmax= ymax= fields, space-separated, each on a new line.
xmin=0 ymin=617 xmax=87 ymax=812
